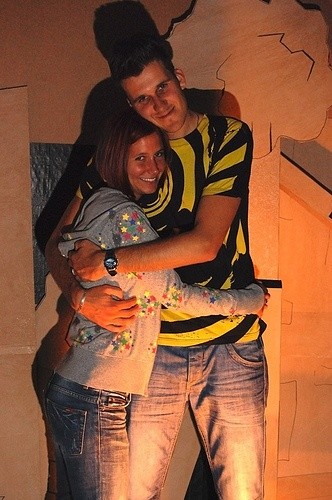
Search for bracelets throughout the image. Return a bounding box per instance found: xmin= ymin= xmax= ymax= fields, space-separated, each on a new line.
xmin=74 ymin=288 xmax=88 ymax=313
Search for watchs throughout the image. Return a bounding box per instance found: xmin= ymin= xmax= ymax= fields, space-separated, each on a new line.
xmin=104 ymin=249 xmax=118 ymax=277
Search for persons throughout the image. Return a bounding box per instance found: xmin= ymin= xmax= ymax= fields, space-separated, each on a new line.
xmin=39 ymin=109 xmax=267 ymax=500
xmin=45 ymin=43 xmax=268 ymax=500
